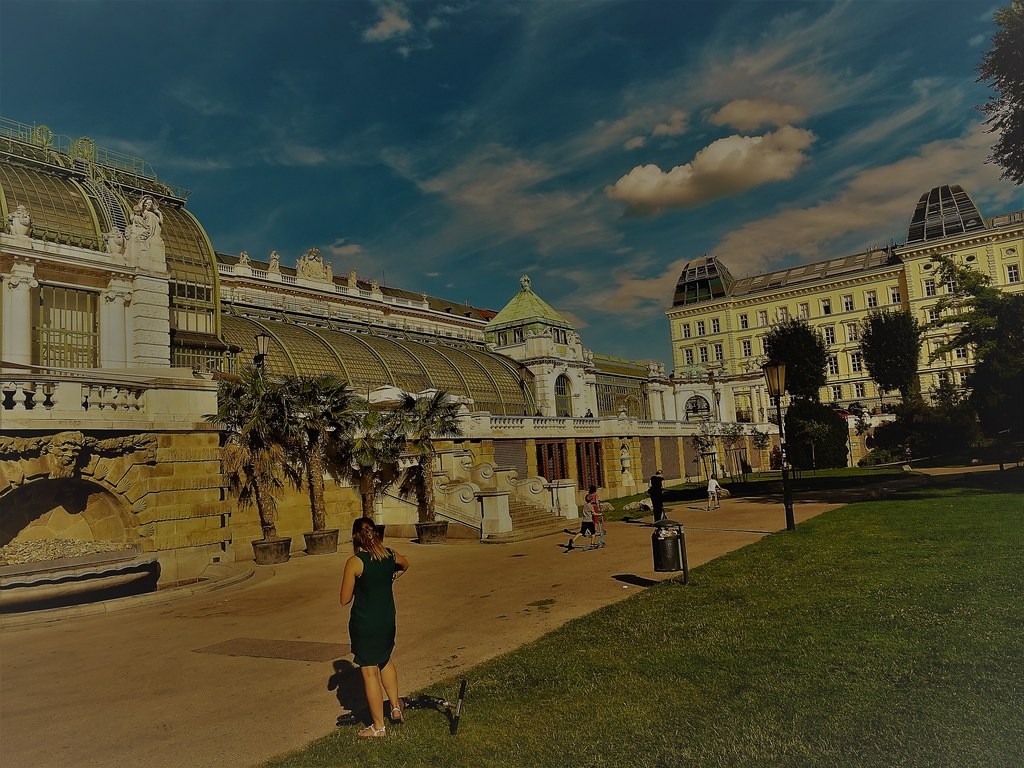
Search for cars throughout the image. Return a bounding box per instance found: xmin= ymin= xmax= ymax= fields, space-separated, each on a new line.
xmin=833 ymin=408 xmax=860 ymax=421
xmin=848 ymin=403 xmax=872 ymax=417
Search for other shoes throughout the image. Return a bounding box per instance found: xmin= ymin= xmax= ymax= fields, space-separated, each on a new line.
xmin=568 ymin=539 xmax=574 ymax=550
xmin=590 ymin=543 xmax=598 ymax=547
xmin=707 ymin=506 xmax=710 ymax=511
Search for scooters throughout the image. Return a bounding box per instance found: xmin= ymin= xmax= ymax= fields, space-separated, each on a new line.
xmin=589 ymin=513 xmax=608 ymax=537
xmin=581 ymin=515 xmax=606 ymax=551
xmin=706 ymin=488 xmax=722 ymax=511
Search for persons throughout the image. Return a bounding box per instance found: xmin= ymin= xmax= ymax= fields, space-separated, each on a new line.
xmin=589 ymin=486 xmax=600 ymax=536
xmin=269 ymin=251 xmax=280 ymax=270
xmin=348 ymin=267 xmax=357 ymax=289
xmin=707 ymin=474 xmax=721 ymax=510
xmin=371 ymin=279 xmax=383 ymax=295
xmin=906 ymin=444 xmax=913 ymax=464
xmin=584 ymin=409 xmax=593 ymax=418
xmin=133 ymin=205 xmax=151 ymax=229
xmin=325 ymin=261 xmax=333 ymax=281
xmin=536 ymin=409 xmax=543 ymax=416
xmin=130 ymin=193 xmax=165 ymax=261
xmin=340 ymin=516 xmax=408 ymax=737
xmin=567 ymin=495 xmax=602 ymax=549
xmin=648 ymin=470 xmax=665 ymax=522
xmin=241 ymin=250 xmax=251 ymax=265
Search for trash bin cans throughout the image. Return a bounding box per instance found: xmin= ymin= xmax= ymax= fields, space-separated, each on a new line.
xmin=649 ymin=520 xmax=681 ymax=572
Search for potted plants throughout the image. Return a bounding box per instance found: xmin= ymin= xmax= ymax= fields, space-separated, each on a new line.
xmin=205 ymin=365 xmax=467 ymax=564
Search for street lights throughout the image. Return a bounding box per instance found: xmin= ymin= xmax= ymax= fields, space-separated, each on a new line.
xmin=639 ymin=379 xmax=647 ymax=419
xmin=984 ymin=388 xmax=1004 ymax=473
xmin=255 ymin=330 xmax=270 ymax=378
xmin=760 ymin=353 xmax=795 ymax=532
xmin=928 ymin=387 xmax=933 ymax=406
xmin=878 ymin=388 xmax=884 ymax=406
xmin=715 ymin=391 xmax=721 ymax=421
xmin=519 ymin=364 xmax=527 ymax=415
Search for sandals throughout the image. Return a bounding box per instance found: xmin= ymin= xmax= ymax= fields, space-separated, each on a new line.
xmin=358 ymin=724 xmax=386 ymax=738
xmin=390 ymin=707 xmax=404 ymax=723
